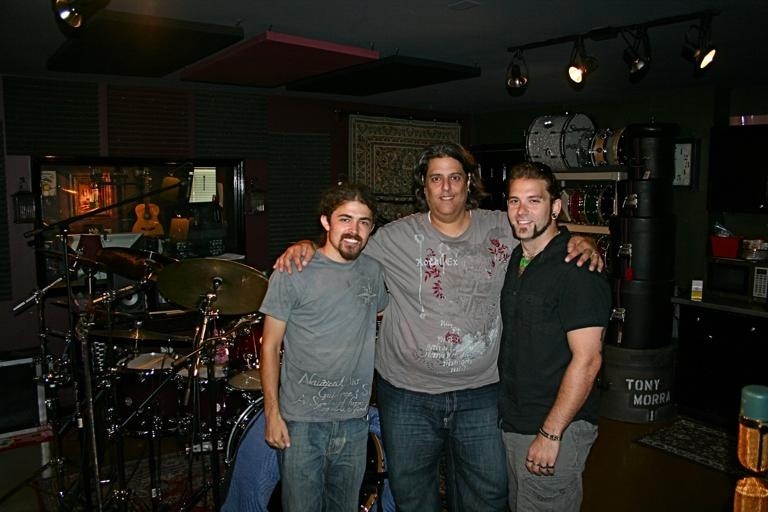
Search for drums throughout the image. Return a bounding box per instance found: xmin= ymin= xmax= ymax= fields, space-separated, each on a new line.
xmin=574 ymin=127 xmax=640 ymax=170
xmin=225 ymin=396 xmax=385 ymax=512
xmin=116 ymin=357 xmax=179 ymax=430
xmin=231 ymin=320 xmax=287 ymax=370
xmin=559 ymin=185 xmax=612 ymax=226
xmin=526 ymin=114 xmax=595 ymax=172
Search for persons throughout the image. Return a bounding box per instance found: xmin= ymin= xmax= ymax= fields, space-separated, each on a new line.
xmin=258 ymin=181 xmax=391 ymax=511
xmin=495 ymin=162 xmax=610 ymax=511
xmin=271 ymin=138 xmax=605 ymax=511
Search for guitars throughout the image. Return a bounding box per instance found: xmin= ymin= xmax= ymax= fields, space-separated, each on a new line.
xmin=132 ymin=168 xmax=166 ymax=237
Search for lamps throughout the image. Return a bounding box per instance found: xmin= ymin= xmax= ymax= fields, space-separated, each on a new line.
xmin=507 ymin=14 xmax=720 ymax=98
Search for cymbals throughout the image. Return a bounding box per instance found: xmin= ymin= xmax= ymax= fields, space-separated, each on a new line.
xmin=154 ymin=257 xmax=269 ymax=315
xmin=88 ymin=328 xmax=177 ymax=343
xmin=47 ymin=299 xmax=131 ymax=320
xmin=99 ymin=246 xmax=180 ymax=284
xmin=230 ymin=371 xmax=282 ymax=390
xmin=39 ymin=249 xmax=103 ymax=271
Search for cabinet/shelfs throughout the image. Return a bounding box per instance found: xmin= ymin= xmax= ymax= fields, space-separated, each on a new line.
xmin=550 ymin=173 xmax=630 ymax=237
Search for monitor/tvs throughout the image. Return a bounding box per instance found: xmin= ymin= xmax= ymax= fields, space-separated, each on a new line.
xmin=706 ymin=124 xmax=768 ymax=214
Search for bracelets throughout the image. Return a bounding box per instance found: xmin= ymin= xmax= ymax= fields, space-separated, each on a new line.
xmin=537 ymin=424 xmax=560 ymax=441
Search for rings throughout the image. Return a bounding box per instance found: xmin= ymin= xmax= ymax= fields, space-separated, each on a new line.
xmin=548 ymin=465 xmax=554 ymax=469
xmin=592 ymin=251 xmax=600 ymax=256
xmin=533 ymin=462 xmax=540 ymax=467
xmin=526 ymin=457 xmax=533 ymax=462
xmin=540 ymin=465 xmax=547 ymax=468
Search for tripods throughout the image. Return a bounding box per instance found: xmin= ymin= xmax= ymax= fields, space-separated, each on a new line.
xmin=178 ymin=361 xmax=230 ymax=512
xmin=95 ymin=305 xmax=153 ymax=511
